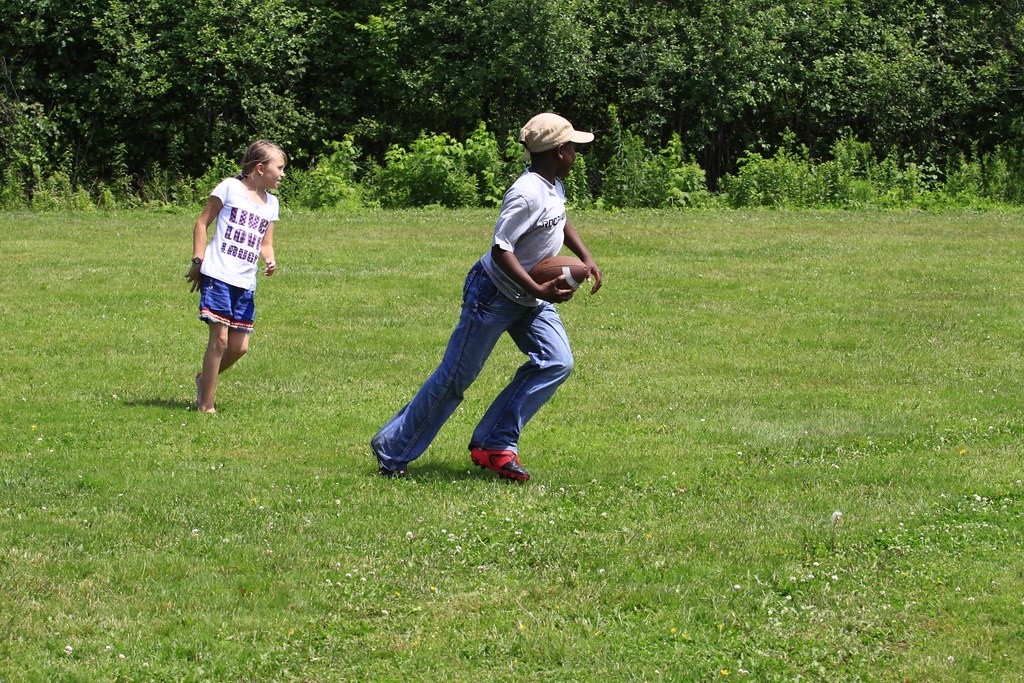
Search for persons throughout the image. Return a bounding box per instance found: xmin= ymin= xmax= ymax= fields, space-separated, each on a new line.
xmin=185 ymin=138 xmax=286 ymax=413
xmin=373 ymin=113 xmax=602 ymax=481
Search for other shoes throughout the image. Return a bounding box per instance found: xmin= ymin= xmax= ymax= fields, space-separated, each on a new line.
xmin=195 ymin=373 xmax=203 ymax=408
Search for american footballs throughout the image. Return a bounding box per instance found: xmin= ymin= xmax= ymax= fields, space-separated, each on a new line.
xmin=529 ymin=256 xmax=588 ymax=297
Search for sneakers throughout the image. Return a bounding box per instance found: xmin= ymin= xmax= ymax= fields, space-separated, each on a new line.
xmin=470 ymin=448 xmax=531 ymax=481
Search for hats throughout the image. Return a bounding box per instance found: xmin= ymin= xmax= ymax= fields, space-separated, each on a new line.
xmin=518 ymin=112 xmax=595 ymax=153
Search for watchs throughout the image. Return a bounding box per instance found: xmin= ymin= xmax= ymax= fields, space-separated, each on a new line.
xmin=191 ymin=257 xmax=203 ymax=264
